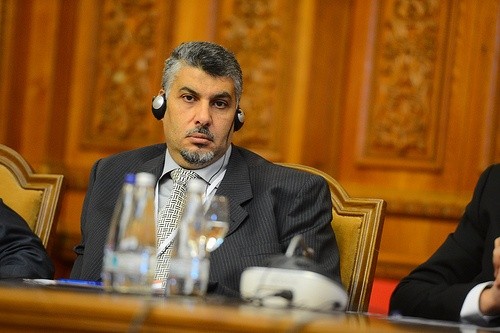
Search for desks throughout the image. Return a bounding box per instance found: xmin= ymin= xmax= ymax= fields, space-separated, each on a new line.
xmin=0 ymin=279 xmax=500 ymax=333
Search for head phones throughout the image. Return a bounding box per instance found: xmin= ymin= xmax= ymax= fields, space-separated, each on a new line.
xmin=151 ymin=94 xmax=245 ymax=131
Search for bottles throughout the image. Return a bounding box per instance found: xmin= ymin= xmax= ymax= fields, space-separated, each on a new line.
xmin=104 ymin=173 xmax=136 ymax=293
xmin=176 ymin=179 xmax=209 ymax=296
xmin=118 ymin=172 xmax=157 ymax=293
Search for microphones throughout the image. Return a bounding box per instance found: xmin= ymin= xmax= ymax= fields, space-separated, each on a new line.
xmin=240 ymin=235 xmax=347 ymax=313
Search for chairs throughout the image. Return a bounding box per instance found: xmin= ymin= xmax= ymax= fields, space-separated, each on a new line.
xmin=0 ymin=144 xmax=65 ymax=250
xmin=274 ymin=162 xmax=387 ymax=313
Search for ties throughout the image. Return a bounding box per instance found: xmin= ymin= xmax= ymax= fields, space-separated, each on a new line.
xmin=153 ymin=166 xmax=200 ymax=297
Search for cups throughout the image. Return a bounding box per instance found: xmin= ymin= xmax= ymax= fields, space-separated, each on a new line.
xmin=204 ymin=197 xmax=230 ymax=253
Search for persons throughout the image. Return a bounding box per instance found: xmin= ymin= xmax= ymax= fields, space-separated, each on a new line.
xmin=0 ymin=198 xmax=55 ymax=279
xmin=69 ymin=41 xmax=341 ymax=299
xmin=388 ymin=164 xmax=500 ymax=326
